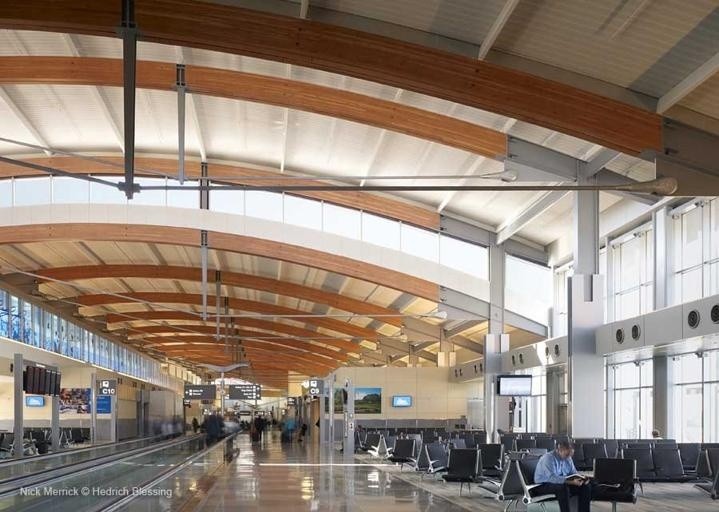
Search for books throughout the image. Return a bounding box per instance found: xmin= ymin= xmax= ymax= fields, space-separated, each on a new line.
xmin=565 ymin=473 xmax=593 ymax=480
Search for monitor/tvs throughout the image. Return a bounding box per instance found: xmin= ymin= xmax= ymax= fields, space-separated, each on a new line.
xmin=393 ymin=396 xmax=411 ymax=407
xmin=26 ymin=396 xmax=44 ymax=406
xmin=499 ymin=375 xmax=532 ymax=396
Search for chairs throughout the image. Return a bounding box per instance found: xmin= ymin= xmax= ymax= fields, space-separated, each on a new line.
xmin=0 ymin=427 xmax=91 ymax=459
xmin=354 ymin=423 xmax=719 ymax=512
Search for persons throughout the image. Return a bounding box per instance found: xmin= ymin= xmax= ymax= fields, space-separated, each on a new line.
xmin=533 ymin=438 xmax=593 ymax=510
xmin=190 ymin=410 xmax=308 ymax=448
xmin=650 ymin=429 xmax=663 ymax=439
